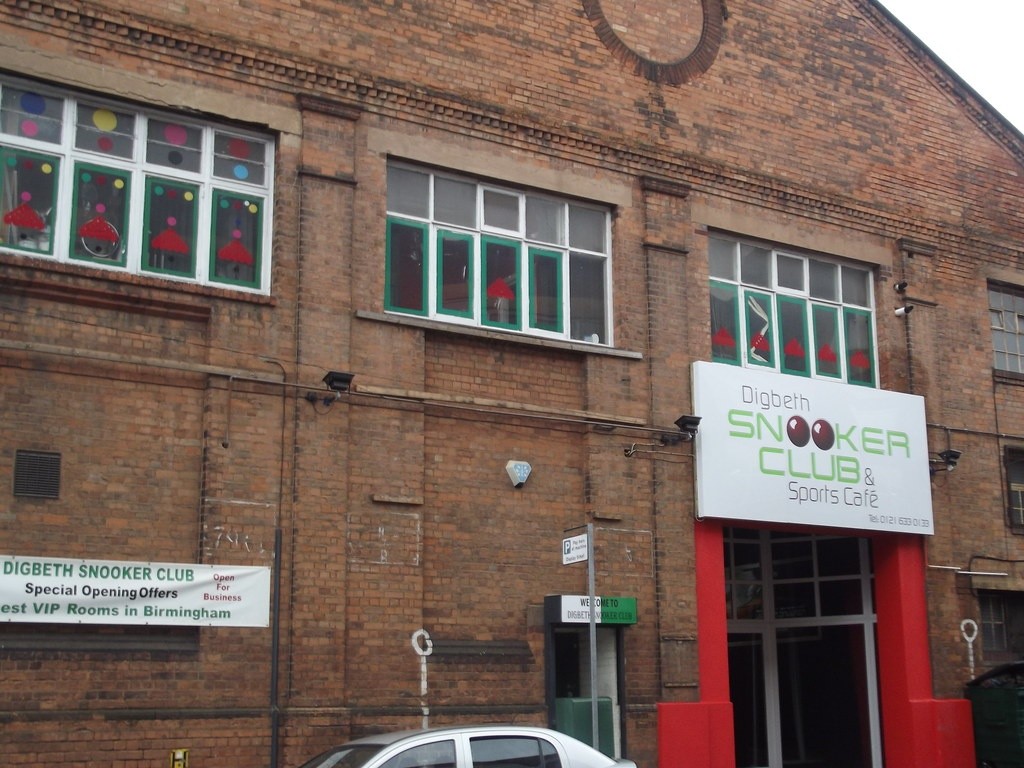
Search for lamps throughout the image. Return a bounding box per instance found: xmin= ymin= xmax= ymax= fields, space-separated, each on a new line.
xmin=671 ymin=415 xmax=703 ymax=445
xmin=928 ymin=448 xmax=962 ymax=472
xmin=321 ymin=371 xmax=356 ymax=407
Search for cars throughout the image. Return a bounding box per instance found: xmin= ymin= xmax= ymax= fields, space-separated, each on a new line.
xmin=298 ymin=724 xmax=639 ymax=768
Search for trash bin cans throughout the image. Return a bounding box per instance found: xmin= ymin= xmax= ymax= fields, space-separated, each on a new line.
xmin=963 ymin=661 xmax=1024 ymax=768
